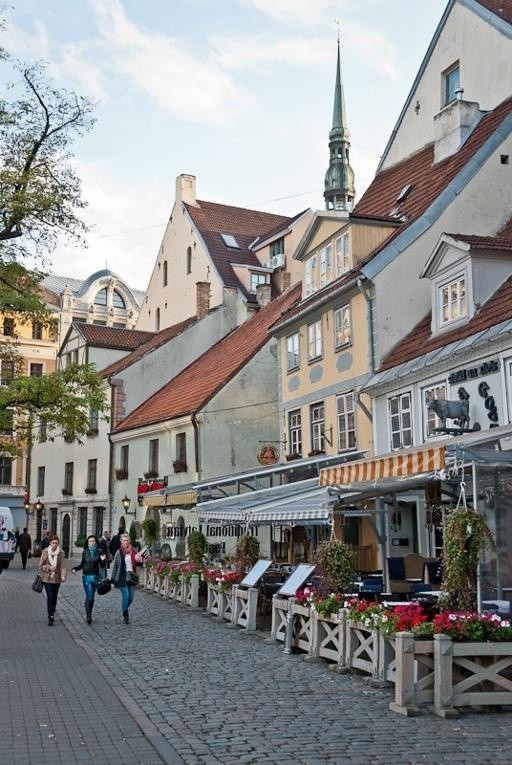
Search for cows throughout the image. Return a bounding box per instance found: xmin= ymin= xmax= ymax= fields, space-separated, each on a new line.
xmin=425 ymin=396 xmax=470 ymax=429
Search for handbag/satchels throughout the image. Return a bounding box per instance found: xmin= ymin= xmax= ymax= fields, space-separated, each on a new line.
xmin=98 ymin=579 xmax=111 ymax=595
xmin=126 ymin=571 xmax=139 ymax=585
xmin=32 ymin=575 xmax=43 ymax=593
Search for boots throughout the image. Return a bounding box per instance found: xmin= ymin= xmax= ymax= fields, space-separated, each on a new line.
xmin=86 ymin=602 xmax=92 ymax=623
xmin=48 ymin=612 xmax=54 ymax=625
xmin=123 ymin=610 xmax=128 ymax=624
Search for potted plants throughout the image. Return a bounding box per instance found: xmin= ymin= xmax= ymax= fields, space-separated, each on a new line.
xmin=432 ymin=508 xmax=498 ymax=613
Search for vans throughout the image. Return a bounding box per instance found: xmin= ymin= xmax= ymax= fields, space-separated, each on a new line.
xmin=0 ymin=506 xmax=18 ymax=569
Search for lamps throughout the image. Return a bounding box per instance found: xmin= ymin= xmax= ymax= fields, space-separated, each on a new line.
xmin=121 ymin=494 xmax=136 ymax=519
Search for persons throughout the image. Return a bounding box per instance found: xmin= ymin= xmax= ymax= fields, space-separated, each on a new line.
xmin=38 ymin=536 xmax=66 ymax=625
xmin=15 ymin=525 xmax=128 ymax=570
xmin=111 ymin=534 xmax=146 ymax=625
xmin=71 ymin=535 xmax=107 ymax=624
